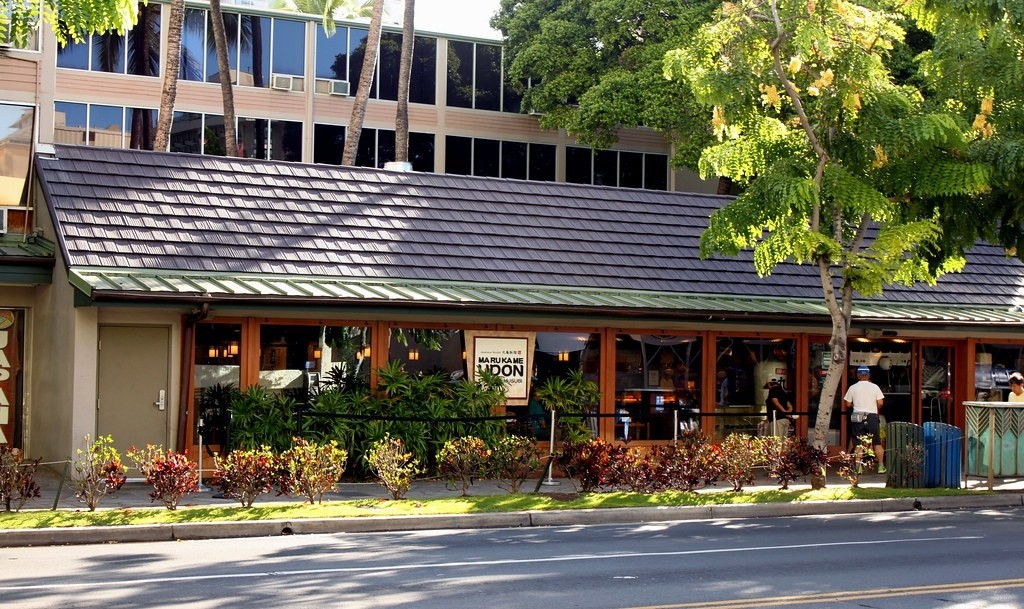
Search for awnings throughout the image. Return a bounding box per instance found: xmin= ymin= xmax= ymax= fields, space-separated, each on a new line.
xmin=70 ymin=264 xmax=1024 ymax=340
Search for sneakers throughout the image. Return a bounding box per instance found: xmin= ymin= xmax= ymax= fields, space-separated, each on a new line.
xmin=878 ymin=466 xmax=887 ymax=474
xmin=857 ymin=467 xmax=863 ymax=475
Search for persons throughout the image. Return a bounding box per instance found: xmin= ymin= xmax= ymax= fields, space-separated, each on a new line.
xmin=1008 ymin=372 xmax=1024 ymax=402
xmin=716 ymin=369 xmax=818 ymax=438
xmin=844 ymin=365 xmax=887 ymax=473
xmin=530 ymin=393 xmax=547 ymax=441
xmin=762 ymin=376 xmax=794 ymax=441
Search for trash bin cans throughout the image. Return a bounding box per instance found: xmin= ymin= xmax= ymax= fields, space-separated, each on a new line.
xmin=922 ymin=422 xmax=962 ymax=489
xmin=886 ymin=422 xmax=925 ymax=489
xmin=962 ymin=401 xmax=1024 ymax=492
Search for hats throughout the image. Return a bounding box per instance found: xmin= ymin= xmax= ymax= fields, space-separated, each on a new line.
xmin=763 ymin=376 xmax=778 ymax=389
xmin=857 ymin=365 xmax=870 ymax=374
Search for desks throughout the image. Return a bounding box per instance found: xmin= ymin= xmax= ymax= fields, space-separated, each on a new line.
xmin=615 ymin=421 xmax=649 ymax=441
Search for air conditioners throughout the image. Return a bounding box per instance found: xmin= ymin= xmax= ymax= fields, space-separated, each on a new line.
xmin=327 ymin=80 xmax=351 ymax=97
xmin=272 ymin=75 xmax=292 ymax=91
